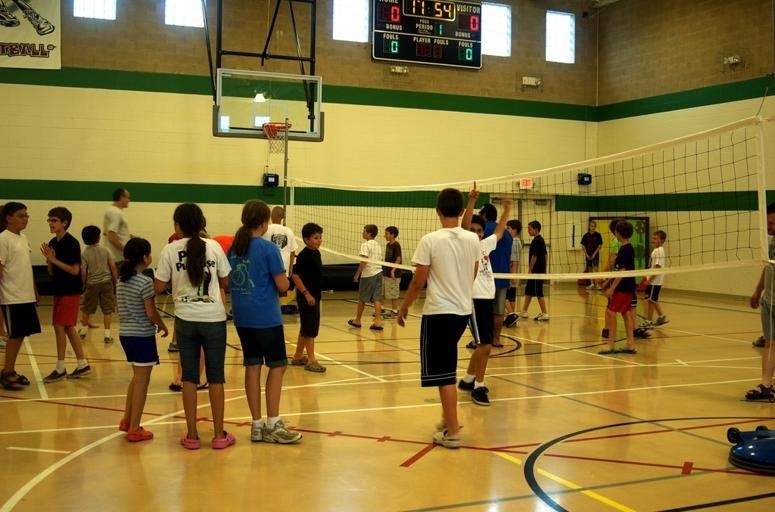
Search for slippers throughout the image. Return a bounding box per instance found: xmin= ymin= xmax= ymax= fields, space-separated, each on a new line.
xmin=369 ymin=323 xmax=384 ymax=330
xmin=491 ymin=342 xmax=504 ymax=348
xmin=169 ymin=383 xmax=182 ymax=392
xmin=210 ymin=430 xmax=237 ymax=450
xmin=465 ymin=341 xmax=477 ymax=349
xmin=347 ymin=319 xmax=361 ymax=328
xmin=196 ymin=382 xmax=209 ymax=391
xmin=179 ymin=435 xmax=202 ymax=451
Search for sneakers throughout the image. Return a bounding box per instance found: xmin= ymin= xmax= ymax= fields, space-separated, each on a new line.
xmin=515 ymin=311 xmax=529 ymax=318
xmin=304 ymin=360 xmax=326 ymax=372
xmin=292 ymin=356 xmax=309 ymax=365
xmin=458 ymin=378 xmax=474 ymax=391
xmin=382 ymin=309 xmax=400 ymax=320
xmin=432 ymin=428 xmax=463 ymax=449
xmin=637 ymin=319 xmax=653 ymax=328
xmin=103 ymin=336 xmax=115 ymax=345
xmin=433 ymin=422 xmax=450 ymax=433
xmin=470 ymin=386 xmax=491 ymax=406
xmin=77 ymin=328 xmax=89 ymax=341
xmin=167 ymin=342 xmax=179 ymax=352
xmin=506 ymin=314 xmax=521 ymax=328
xmin=43 ymin=368 xmax=68 ymax=383
xmin=66 ymin=365 xmax=93 ymax=379
xmin=251 ymin=421 xmax=265 ymax=441
xmin=596 ymin=284 xmax=602 ymax=290
xmin=653 ymin=314 xmax=670 ymax=326
xmin=751 ymin=336 xmax=766 ymax=346
xmin=533 ymin=312 xmax=549 ymax=320
xmin=373 ymin=309 xmax=384 ymax=318
xmin=262 ymin=420 xmax=304 ymax=445
xmin=585 ymin=284 xmax=595 ymax=290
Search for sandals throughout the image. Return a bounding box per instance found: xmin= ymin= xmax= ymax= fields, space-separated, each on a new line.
xmin=0 ymin=368 xmax=24 ymax=391
xmin=615 ymin=348 xmax=637 ymax=354
xmin=744 ymin=382 xmax=774 ymax=402
xmin=119 ymin=418 xmax=129 ymax=432
xmin=633 ymin=328 xmax=652 ymax=339
xmin=127 ymin=426 xmax=154 ymax=443
xmin=13 ymin=370 xmax=31 ymax=385
xmin=601 ymin=327 xmax=609 ymax=337
xmin=598 ymin=349 xmax=617 ymax=354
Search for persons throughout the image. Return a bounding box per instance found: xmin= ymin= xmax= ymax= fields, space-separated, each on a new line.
xmin=117 ymin=237 xmax=168 ymax=442
xmin=601 ymin=221 xmax=637 ymax=356
xmin=261 ymin=204 xmax=295 ymax=281
xmin=79 ymin=226 xmax=118 ymax=344
xmin=742 ymin=199 xmax=775 ymax=404
xmin=504 ymin=219 xmax=520 ymax=329
xmin=40 ymin=206 xmax=92 ymax=383
xmin=397 ymin=186 xmax=479 ymax=448
xmin=226 ymin=199 xmax=303 ymax=445
xmin=155 ymin=203 xmax=233 ymax=450
xmin=458 ymin=180 xmax=511 ymax=405
xmin=346 ymin=224 xmax=385 ymax=331
xmin=603 ymin=217 xmax=651 ymax=338
xmin=580 ymin=219 xmax=603 ymax=290
xmin=0 ymin=202 xmax=42 ymax=391
xmin=292 ymin=222 xmax=326 ymax=373
xmin=103 ymin=188 xmax=138 ymax=277
xmin=465 ymin=202 xmax=512 ymax=348
xmin=520 ymin=221 xmax=549 ymax=322
xmin=640 ymin=230 xmax=670 ymax=329
xmin=381 ymin=225 xmax=403 ymax=321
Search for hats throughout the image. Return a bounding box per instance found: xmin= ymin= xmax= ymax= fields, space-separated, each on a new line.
xmin=478 ymin=203 xmax=498 ymax=218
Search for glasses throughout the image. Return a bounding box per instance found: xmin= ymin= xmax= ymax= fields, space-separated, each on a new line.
xmin=8 ymin=213 xmax=29 ymax=220
xmin=46 ymin=218 xmax=63 ymax=224
